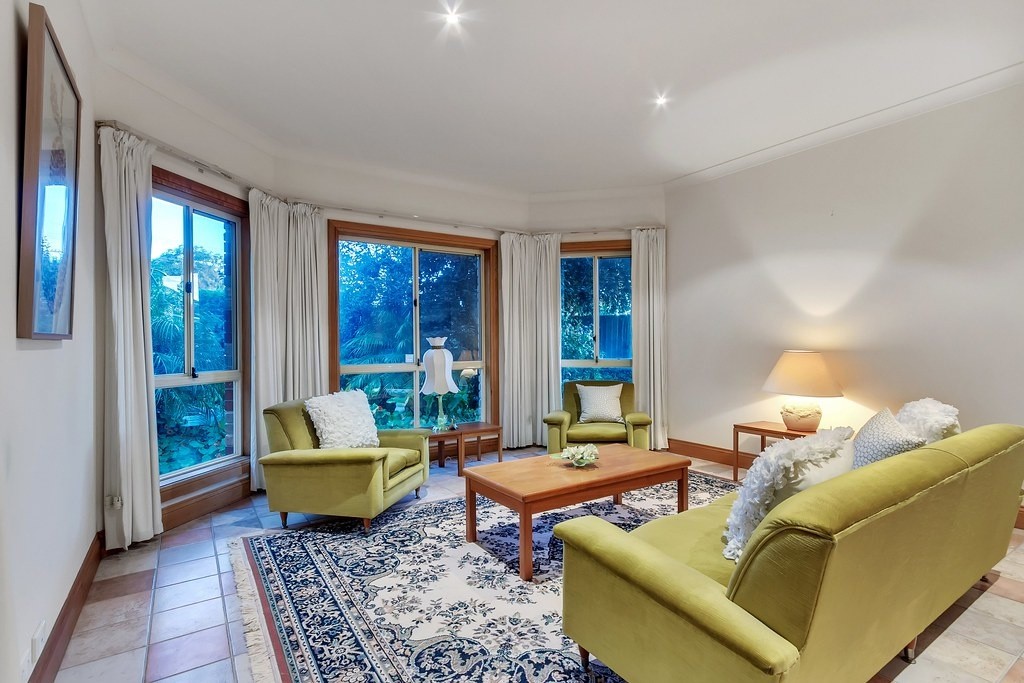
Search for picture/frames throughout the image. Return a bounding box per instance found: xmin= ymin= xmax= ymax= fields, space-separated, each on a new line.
xmin=15 ymin=1 xmax=85 ymax=342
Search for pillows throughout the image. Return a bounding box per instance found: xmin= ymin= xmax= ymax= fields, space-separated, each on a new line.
xmin=306 ymin=389 xmax=379 ymax=449
xmin=852 ymin=407 xmax=926 ymax=473
xmin=893 ymin=397 xmax=962 ymax=445
xmin=575 ymin=383 xmax=625 ymax=424
xmin=718 ymin=427 xmax=855 ymax=566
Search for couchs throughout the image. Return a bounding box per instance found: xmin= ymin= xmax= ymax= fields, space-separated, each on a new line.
xmin=256 ymin=395 xmax=430 ymax=535
xmin=542 ymin=379 xmax=653 ymax=454
xmin=551 ymin=420 xmax=1024 ymax=682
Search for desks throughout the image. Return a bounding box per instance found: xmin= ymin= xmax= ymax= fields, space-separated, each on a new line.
xmin=734 ymin=421 xmax=827 ymax=484
xmin=418 ymin=421 xmax=505 ymax=477
xmin=463 ymin=443 xmax=692 ymax=582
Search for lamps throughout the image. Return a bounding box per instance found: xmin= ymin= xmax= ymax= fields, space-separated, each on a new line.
xmin=419 ymin=335 xmax=460 ymax=433
xmin=762 ymin=348 xmax=845 ymax=433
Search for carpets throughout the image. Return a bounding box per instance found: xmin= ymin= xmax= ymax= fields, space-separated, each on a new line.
xmin=225 ymin=470 xmax=740 ymax=683
